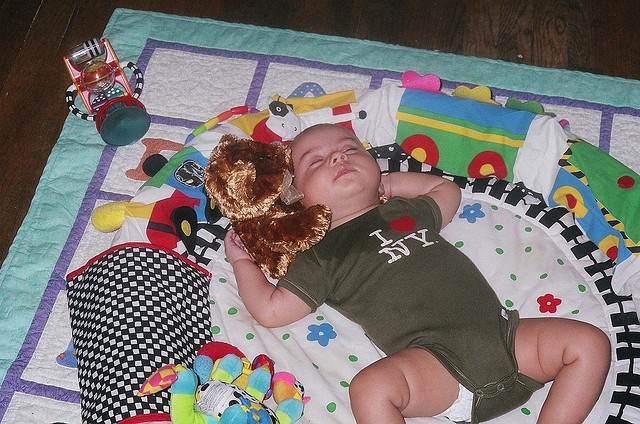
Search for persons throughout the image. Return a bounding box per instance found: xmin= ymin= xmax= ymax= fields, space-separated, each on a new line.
xmin=224 ymin=125 xmax=610 ymax=424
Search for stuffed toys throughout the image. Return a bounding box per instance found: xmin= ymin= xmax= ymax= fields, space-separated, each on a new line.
xmin=203 ymin=133 xmax=332 ymax=279
